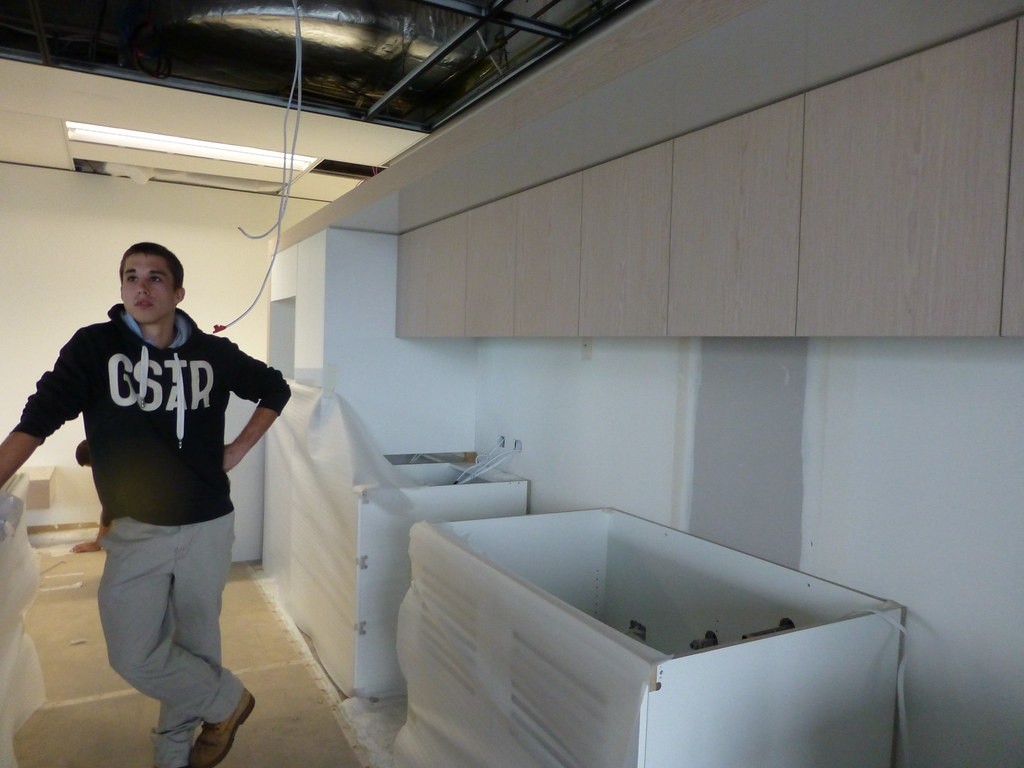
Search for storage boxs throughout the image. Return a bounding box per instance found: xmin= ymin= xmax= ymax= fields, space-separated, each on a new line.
xmin=262 ymin=452 xmax=902 ymax=768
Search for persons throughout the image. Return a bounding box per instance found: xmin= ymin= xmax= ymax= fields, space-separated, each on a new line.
xmin=71 ymin=438 xmax=112 ymax=554
xmin=1 ymin=240 xmax=294 ymax=767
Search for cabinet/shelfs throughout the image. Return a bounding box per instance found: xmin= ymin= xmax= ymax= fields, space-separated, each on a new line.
xmin=397 ymin=16 xmax=1024 ymax=336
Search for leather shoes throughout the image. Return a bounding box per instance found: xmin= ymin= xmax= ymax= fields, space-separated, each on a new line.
xmin=190 ymin=688 xmax=255 ymax=768
